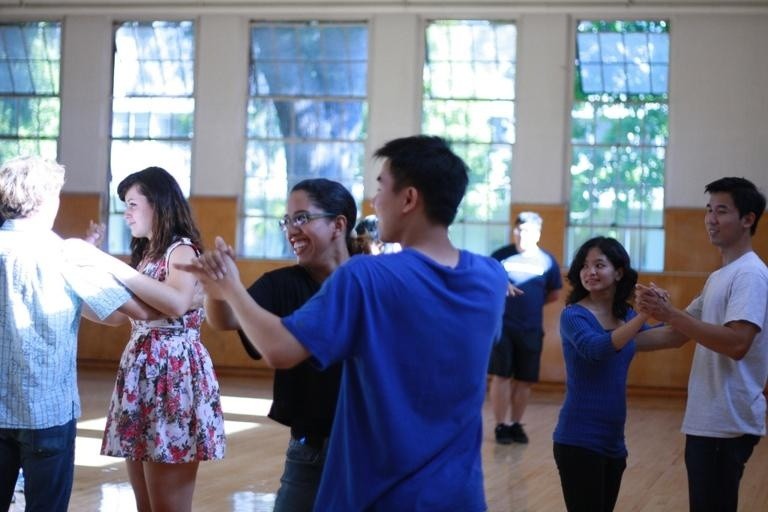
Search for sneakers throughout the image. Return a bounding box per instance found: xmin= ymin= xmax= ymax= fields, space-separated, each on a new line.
xmin=494 ymin=422 xmax=527 ymax=444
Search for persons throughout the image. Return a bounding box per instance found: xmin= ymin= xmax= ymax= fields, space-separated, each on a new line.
xmin=620 ymin=170 xmax=768 ymax=511
xmin=477 ymin=209 xmax=565 ymax=445
xmin=548 ymin=235 xmax=675 ymax=511
xmin=0 ymin=159 xmax=158 ymax=511
xmin=90 ymin=165 xmax=228 ymax=512
xmin=189 ymin=131 xmax=504 ymax=512
xmin=170 ymin=176 xmax=526 ymax=512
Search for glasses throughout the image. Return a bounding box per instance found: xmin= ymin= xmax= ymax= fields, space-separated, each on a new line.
xmin=279 ymin=211 xmax=335 ymax=231
